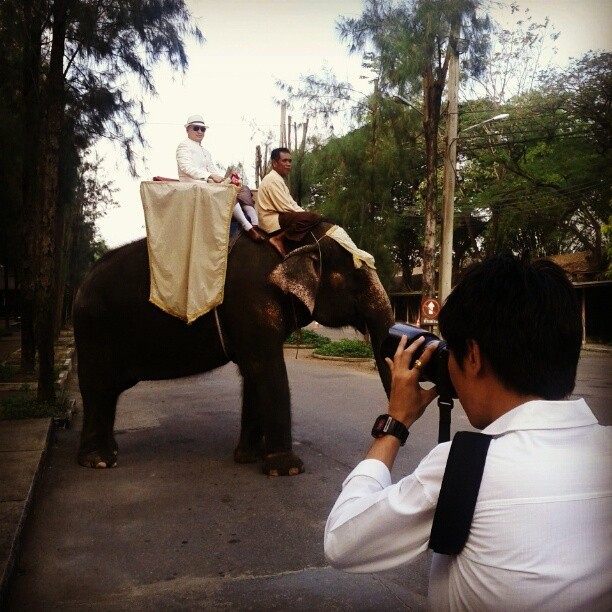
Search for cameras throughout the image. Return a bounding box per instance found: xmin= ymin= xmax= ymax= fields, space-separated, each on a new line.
xmin=379 ymin=321 xmax=457 ymax=401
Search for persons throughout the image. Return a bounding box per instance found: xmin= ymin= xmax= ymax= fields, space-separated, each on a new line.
xmin=175 ymin=113 xmax=270 ymax=244
xmin=322 ymin=258 xmax=612 ymax=612
xmin=254 ymin=148 xmax=322 ymax=257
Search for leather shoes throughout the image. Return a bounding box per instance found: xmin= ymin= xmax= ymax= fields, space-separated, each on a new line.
xmin=248 ymin=228 xmax=268 ymax=243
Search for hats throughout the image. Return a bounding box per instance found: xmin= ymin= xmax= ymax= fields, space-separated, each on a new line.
xmin=184 ymin=115 xmax=209 ymax=129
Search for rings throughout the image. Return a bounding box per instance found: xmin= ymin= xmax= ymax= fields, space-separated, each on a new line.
xmin=414 ymin=359 xmax=424 ymax=369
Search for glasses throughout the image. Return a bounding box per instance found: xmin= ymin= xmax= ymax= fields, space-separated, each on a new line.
xmin=193 ymin=126 xmax=206 ymax=132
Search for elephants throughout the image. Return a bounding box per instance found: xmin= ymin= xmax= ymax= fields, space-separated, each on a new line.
xmin=69 ymin=210 xmax=413 ymax=478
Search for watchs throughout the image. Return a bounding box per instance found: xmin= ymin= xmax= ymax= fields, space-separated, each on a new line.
xmin=370 ymin=413 xmax=410 ymax=446
